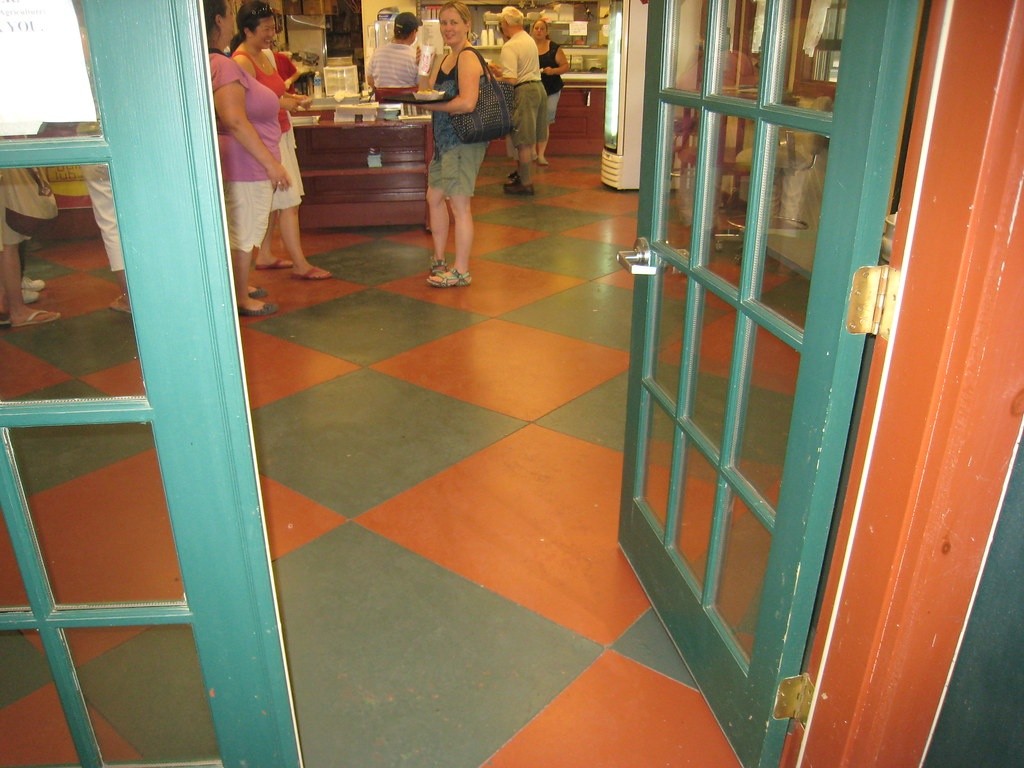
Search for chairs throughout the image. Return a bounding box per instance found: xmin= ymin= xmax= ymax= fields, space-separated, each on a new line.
xmin=714 ymin=97 xmax=834 ymax=251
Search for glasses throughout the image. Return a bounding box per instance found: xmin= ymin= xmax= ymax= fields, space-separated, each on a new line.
xmin=248 ymin=4 xmax=274 ymax=16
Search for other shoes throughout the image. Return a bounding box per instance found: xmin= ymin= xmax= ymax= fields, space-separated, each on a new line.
xmin=248 ymin=285 xmax=268 ymax=298
xmin=236 ymin=304 xmax=277 ymax=317
xmin=108 ymin=293 xmax=132 ymax=314
xmin=531 ymin=153 xmax=549 ymax=166
xmin=507 ymin=171 xmax=520 ymax=181
xmin=504 ymin=177 xmax=534 ymax=195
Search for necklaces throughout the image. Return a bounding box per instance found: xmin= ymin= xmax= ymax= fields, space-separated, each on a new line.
xmin=248 ymin=50 xmax=265 ymax=67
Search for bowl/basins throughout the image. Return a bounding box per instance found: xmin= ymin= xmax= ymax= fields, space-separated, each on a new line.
xmin=292 ymin=116 xmax=319 ymax=125
xmin=412 ymin=90 xmax=446 ymax=101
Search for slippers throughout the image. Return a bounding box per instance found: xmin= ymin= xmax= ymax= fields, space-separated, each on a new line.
xmin=256 ymin=258 xmax=294 ymax=269
xmin=0 ymin=309 xmax=62 ymax=327
xmin=292 ymin=265 xmax=333 ymax=279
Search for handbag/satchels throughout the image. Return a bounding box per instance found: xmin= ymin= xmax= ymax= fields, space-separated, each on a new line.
xmin=447 ymin=46 xmax=514 ymax=143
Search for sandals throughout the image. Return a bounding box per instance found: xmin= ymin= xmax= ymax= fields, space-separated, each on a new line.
xmin=428 ymin=256 xmax=448 ymax=274
xmin=427 ymin=268 xmax=473 ymax=287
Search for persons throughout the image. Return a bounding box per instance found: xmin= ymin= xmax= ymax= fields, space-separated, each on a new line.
xmin=203 ymin=0 xmax=292 ymax=315
xmin=0 ymin=135 xmax=61 ymax=328
xmin=76 ymin=121 xmax=131 ymax=314
xmin=409 ymin=1 xmax=490 ymax=288
xmin=674 ymin=30 xmax=756 ymax=212
xmin=528 ymin=17 xmax=569 ymax=165
xmin=367 ymin=12 xmax=423 ymax=103
xmin=487 ymin=5 xmax=547 ymax=195
xmin=229 ymin=0 xmax=333 ymax=280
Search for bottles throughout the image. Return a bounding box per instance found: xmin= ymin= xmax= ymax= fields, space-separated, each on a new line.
xmin=296 ymin=72 xmax=323 ymax=99
xmin=367 ymin=146 xmax=382 ymax=167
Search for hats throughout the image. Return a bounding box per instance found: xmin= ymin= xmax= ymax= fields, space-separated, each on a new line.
xmin=394 ymin=12 xmax=423 ymax=31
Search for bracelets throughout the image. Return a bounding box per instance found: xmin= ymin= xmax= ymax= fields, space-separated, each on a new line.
xmin=292 ymin=93 xmax=296 ymax=97
xmin=287 ymin=79 xmax=292 ymax=84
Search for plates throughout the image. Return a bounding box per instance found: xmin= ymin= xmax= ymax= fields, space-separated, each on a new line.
xmin=527 ymin=4 xmax=574 ymax=21
xmin=399 ymin=115 xmax=432 ymax=123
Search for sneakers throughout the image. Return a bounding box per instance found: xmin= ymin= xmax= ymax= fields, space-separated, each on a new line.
xmin=20 ymin=288 xmax=39 ymax=305
xmin=21 ymin=277 xmax=45 ymax=291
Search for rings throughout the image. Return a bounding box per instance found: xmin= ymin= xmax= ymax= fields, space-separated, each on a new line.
xmin=278 ymin=181 xmax=281 ymax=184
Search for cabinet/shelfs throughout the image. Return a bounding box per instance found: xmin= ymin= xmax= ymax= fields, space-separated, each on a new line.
xmin=418 ymin=0 xmax=612 ymax=55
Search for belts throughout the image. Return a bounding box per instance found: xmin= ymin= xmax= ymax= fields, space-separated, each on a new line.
xmin=513 ymin=79 xmax=535 ymax=88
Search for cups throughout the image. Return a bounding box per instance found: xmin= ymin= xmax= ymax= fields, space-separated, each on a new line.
xmin=481 ymin=28 xmax=504 ymax=45
xmin=484 ymin=58 xmax=493 ymax=64
xmin=418 ymin=45 xmax=436 ymax=77
